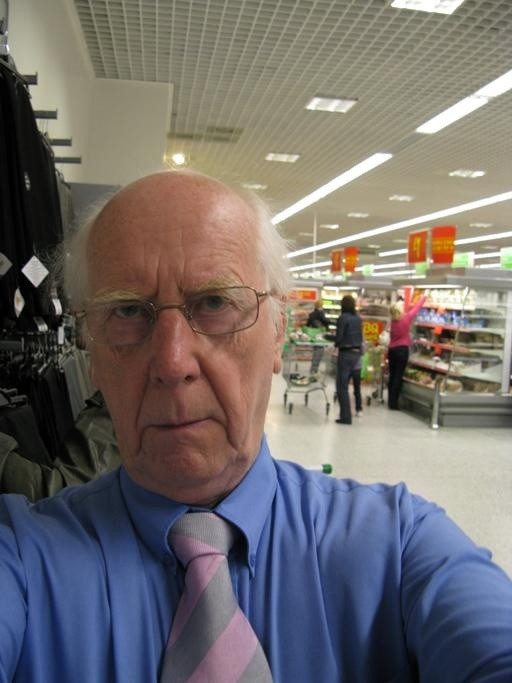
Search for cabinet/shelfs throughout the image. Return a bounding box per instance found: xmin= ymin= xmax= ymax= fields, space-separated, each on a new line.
xmin=391 ymin=277 xmax=512 ymax=429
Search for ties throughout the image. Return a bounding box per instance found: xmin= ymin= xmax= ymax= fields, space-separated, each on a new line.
xmin=161 ymin=512 xmax=273 ymax=683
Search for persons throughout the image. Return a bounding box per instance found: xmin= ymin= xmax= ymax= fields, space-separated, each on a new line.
xmin=323 ymin=296 xmax=368 ymax=424
xmin=388 ymin=288 xmax=430 ymax=410
xmin=307 ymin=302 xmax=328 ymax=382
xmin=0 ymin=168 xmax=512 ymax=683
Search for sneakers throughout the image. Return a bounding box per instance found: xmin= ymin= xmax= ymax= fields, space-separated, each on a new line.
xmin=356 ymin=406 xmax=362 ymax=411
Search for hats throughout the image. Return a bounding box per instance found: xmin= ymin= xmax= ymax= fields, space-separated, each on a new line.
xmin=315 ymin=300 xmax=323 ymax=307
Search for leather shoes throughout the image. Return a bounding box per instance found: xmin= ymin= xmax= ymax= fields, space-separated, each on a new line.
xmin=336 ymin=419 xmax=351 ymax=423
xmin=389 ymin=405 xmax=400 ymax=410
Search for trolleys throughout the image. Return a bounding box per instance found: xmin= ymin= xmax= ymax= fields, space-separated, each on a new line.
xmin=284 ymin=341 xmax=383 ymax=415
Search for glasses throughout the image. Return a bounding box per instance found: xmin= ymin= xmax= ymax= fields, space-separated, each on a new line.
xmin=75 ymin=286 xmax=272 ymax=346
